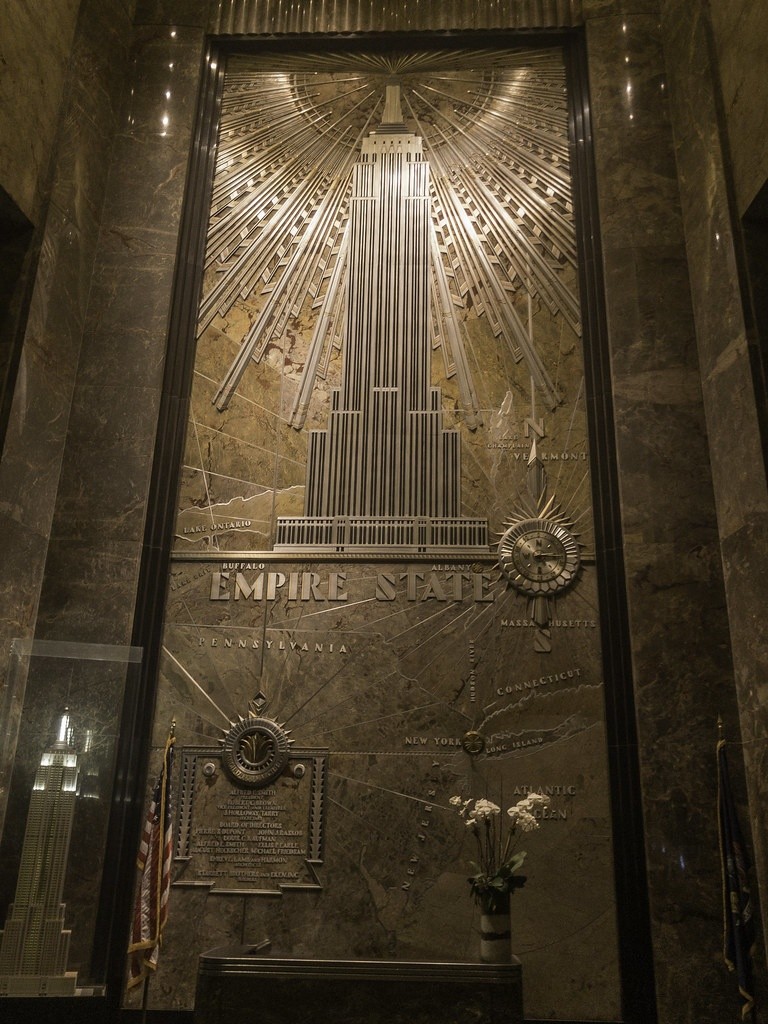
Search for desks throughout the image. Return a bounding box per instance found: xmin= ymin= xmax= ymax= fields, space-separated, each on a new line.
xmin=192 ymin=953 xmax=525 ymax=1024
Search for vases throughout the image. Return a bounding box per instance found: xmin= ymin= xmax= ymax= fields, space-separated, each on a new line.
xmin=479 ymin=892 xmax=512 ymax=964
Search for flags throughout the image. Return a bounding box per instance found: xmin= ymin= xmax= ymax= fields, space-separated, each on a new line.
xmin=715 ymin=737 xmax=767 ymax=1023
xmin=123 ymin=713 xmax=177 ymax=992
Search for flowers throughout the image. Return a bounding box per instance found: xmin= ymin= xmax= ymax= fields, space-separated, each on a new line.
xmin=446 ymin=774 xmax=553 ymax=913
